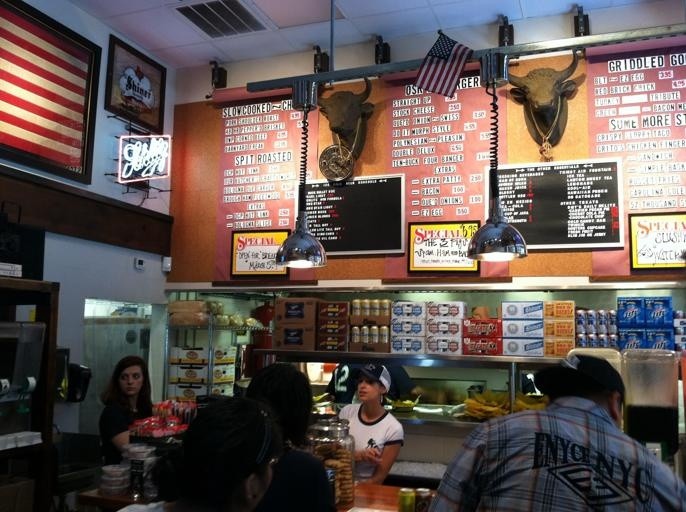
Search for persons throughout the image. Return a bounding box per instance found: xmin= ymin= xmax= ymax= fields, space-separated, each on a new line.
xmin=98 ymin=355 xmax=159 ymax=461
xmin=339 ymin=363 xmax=403 ymax=486
xmin=113 ymin=394 xmax=283 ymax=512
xmin=427 ymin=354 xmax=685 ymax=511
xmin=166 ymin=363 xmax=334 ymax=512
xmin=322 ymin=359 xmax=423 ymax=405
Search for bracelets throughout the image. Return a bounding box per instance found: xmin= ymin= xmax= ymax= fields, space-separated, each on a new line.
xmin=361 ymin=452 xmax=367 ymax=463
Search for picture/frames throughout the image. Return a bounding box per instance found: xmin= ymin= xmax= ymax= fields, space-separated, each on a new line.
xmin=0 ymin=0 xmax=102 ymax=186
xmin=229 ymin=228 xmax=291 ymax=280
xmin=409 ymin=220 xmax=479 ymax=275
xmin=627 ymin=211 xmax=686 ymax=272
xmin=104 ymin=33 xmax=167 ymax=135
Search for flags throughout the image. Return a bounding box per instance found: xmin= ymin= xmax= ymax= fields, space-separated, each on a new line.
xmin=416 ymin=29 xmax=473 ymax=98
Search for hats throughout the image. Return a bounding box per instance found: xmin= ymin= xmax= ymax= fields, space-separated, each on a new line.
xmin=351 ymin=362 xmax=391 ymax=391
xmin=534 ymin=356 xmax=623 ymax=395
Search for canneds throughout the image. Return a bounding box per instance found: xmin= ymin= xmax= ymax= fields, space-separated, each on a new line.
xmin=576 ymin=309 xmax=619 ymax=350
xmin=673 ymin=311 xmax=686 ymax=350
xmin=399 ymin=487 xmax=416 ymax=512
xmin=414 ymin=488 xmax=432 ymax=512
xmin=350 ymin=299 xmax=391 ymax=343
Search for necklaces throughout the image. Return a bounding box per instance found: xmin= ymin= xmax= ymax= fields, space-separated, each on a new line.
xmin=129 ymin=406 xmax=140 ymax=411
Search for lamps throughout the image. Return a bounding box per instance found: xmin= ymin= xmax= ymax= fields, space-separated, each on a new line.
xmin=275 ymin=78 xmax=327 ymax=269
xmin=467 ymin=50 xmax=529 ymax=262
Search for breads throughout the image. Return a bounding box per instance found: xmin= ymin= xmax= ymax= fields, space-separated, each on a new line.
xmin=168 ymin=300 xmax=208 ymax=327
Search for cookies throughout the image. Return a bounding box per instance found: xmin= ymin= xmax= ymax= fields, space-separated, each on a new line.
xmin=312 ymin=444 xmax=354 ymax=504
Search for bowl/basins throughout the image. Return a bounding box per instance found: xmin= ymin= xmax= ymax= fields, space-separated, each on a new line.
xmin=97 ymin=444 xmax=158 ymax=499
